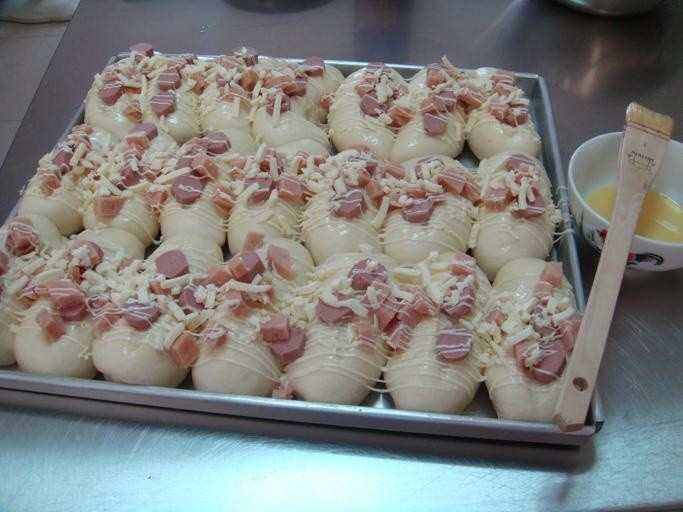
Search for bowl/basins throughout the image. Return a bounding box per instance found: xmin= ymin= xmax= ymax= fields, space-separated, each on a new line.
xmin=569 ymin=131 xmax=683 ymax=272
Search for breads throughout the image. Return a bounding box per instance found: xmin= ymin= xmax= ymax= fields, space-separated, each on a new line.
xmin=0 ymin=42 xmax=581 ymax=423
